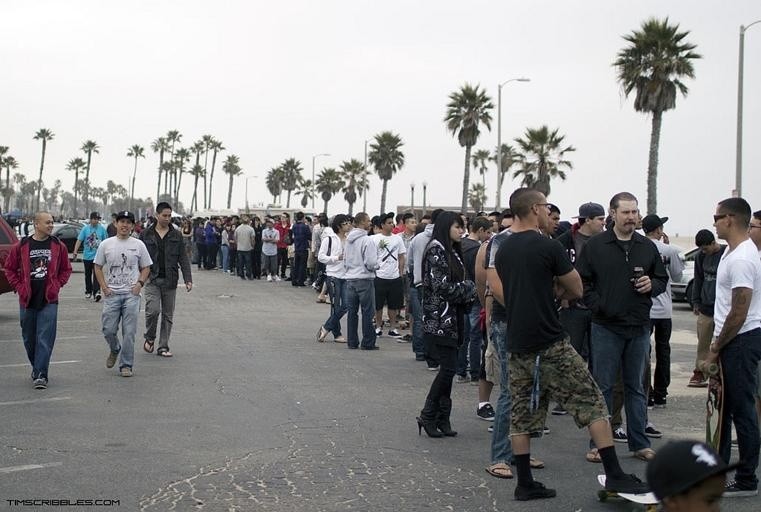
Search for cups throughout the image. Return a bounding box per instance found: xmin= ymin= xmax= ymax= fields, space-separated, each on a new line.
xmin=634 ymin=266 xmax=644 ymax=290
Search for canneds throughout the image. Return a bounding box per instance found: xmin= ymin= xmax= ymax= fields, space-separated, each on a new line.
xmin=632 ymin=267 xmax=644 ymax=293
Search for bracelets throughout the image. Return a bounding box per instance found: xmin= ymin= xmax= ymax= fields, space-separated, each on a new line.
xmin=137 ymin=279 xmax=145 ymax=287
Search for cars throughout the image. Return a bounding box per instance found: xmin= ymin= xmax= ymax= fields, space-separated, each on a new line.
xmin=0 ymin=208 xmax=106 ymax=256
xmin=667 ymin=244 xmax=727 ymax=307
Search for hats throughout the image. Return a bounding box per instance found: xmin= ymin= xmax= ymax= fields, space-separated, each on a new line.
xmin=116 ymin=210 xmax=135 ymax=224
xmin=646 ymin=439 xmax=747 ymax=502
xmin=314 ymin=212 xmax=327 ymax=219
xmin=570 ymin=201 xmax=606 ymax=219
xmin=88 ymin=211 xmax=101 ymax=220
xmin=378 ymin=212 xmax=395 ymax=223
xmin=641 ymin=214 xmax=669 ymax=233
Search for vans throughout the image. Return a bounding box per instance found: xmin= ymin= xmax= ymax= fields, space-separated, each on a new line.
xmin=0 ymin=214 xmax=20 ymax=296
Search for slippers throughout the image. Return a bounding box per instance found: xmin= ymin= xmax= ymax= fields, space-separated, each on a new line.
xmin=144 ymin=339 xmax=155 ymax=353
xmin=485 ymin=452 xmax=545 ymax=479
xmin=584 ymin=445 xmax=657 ymax=463
xmin=157 ymin=350 xmax=173 ymax=357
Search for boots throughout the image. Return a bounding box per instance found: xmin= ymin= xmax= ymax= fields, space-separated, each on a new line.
xmin=438 ymin=398 xmax=462 ymax=438
xmin=416 ymin=398 xmax=446 ymax=439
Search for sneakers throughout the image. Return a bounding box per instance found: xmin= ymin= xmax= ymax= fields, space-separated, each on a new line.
xmin=411 ymin=355 xmax=482 ymax=387
xmin=119 ymin=365 xmax=134 ymax=378
xmin=604 ymin=470 xmax=655 ymax=494
xmin=514 ymin=480 xmax=557 ymax=501
xmin=314 ymin=296 xmax=413 ymax=352
xmin=84 ymin=292 xmax=92 ymax=298
xmin=688 ymin=370 xmax=709 ymax=386
xmin=477 ymin=397 xmax=665 ymax=443
xmin=721 ymin=478 xmax=759 ymax=498
xmin=93 ymin=293 xmax=101 ymax=302
xmin=106 ymin=343 xmax=121 ymax=368
xmin=31 ymin=378 xmax=49 ymax=391
xmin=198 ymin=266 xmax=322 ymax=293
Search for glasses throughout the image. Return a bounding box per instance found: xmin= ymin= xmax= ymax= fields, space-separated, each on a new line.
xmin=713 ymin=213 xmax=736 ymax=223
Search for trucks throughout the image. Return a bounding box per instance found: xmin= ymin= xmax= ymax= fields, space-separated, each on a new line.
xmin=237 ymin=206 xmax=318 ymax=225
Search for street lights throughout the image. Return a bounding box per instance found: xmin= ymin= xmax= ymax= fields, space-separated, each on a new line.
xmin=497 ymin=76 xmax=531 ymax=210
xmin=409 ymin=181 xmax=418 ymax=217
xmin=421 ymin=178 xmax=428 ymax=217
xmin=312 ymin=153 xmax=331 ymax=208
xmin=243 ymin=174 xmax=258 ymax=207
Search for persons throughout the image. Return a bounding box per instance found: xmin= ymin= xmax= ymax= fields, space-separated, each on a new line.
xmin=91 ymin=211 xmax=153 ymax=378
xmin=107 ymin=212 xmax=119 ymax=239
xmin=131 ymin=211 xmax=315 ymax=290
xmin=3 ymin=211 xmax=72 ymax=390
xmin=72 ymin=211 xmax=109 ymax=304
xmin=138 ymin=202 xmax=193 ymax=357
xmin=0 ymin=207 xmax=106 ymax=238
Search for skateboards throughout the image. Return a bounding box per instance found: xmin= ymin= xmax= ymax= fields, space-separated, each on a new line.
xmin=697 ymin=352 xmax=724 ymax=453
xmin=597 ymin=474 xmax=656 ymax=505
